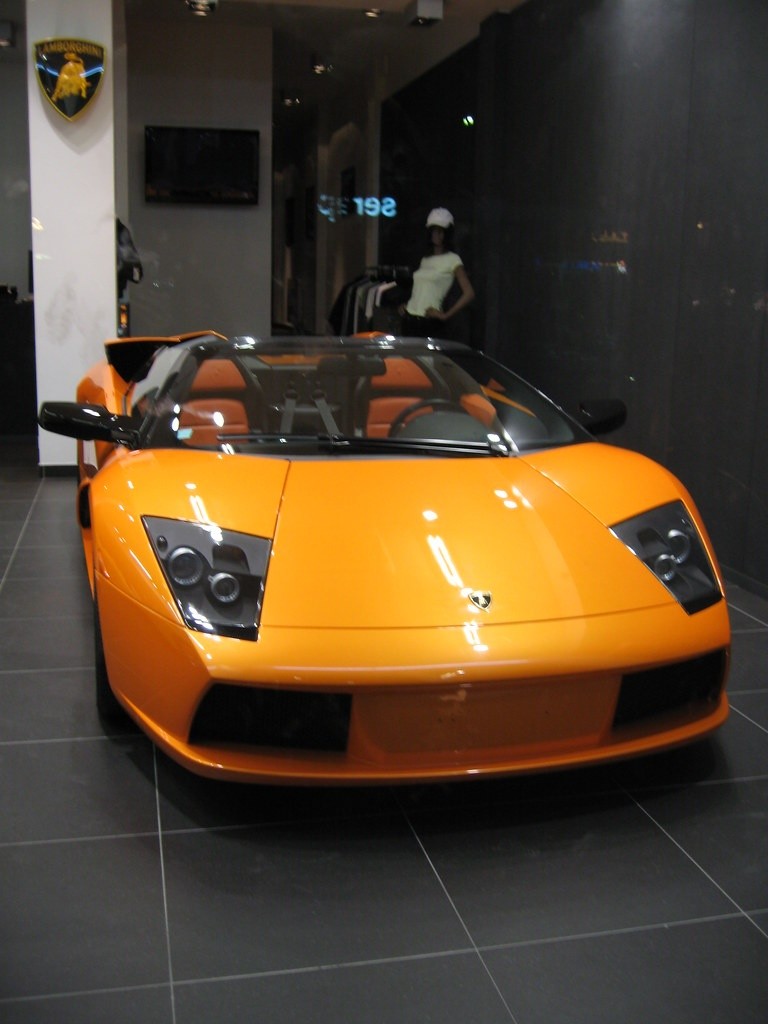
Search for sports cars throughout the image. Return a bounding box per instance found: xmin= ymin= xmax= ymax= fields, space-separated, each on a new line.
xmin=40 ymin=327 xmax=732 ymax=788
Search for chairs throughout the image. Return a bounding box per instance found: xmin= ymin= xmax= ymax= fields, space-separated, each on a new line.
xmin=174 ymin=351 xmax=268 ymax=447
xmin=353 ymin=355 xmax=449 ymax=442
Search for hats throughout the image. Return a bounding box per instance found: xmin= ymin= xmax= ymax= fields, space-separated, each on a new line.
xmin=426 ymin=208 xmax=454 ymax=229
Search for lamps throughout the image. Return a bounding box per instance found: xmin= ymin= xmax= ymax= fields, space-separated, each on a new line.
xmin=408 ymin=0 xmax=442 ymax=26
xmin=184 ymin=0 xmax=217 ymax=16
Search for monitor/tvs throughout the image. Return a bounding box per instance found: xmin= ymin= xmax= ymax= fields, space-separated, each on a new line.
xmin=144 ymin=125 xmax=259 ymax=204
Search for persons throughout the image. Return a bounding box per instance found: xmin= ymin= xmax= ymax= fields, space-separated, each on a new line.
xmin=398 ymin=207 xmax=476 ymax=338
